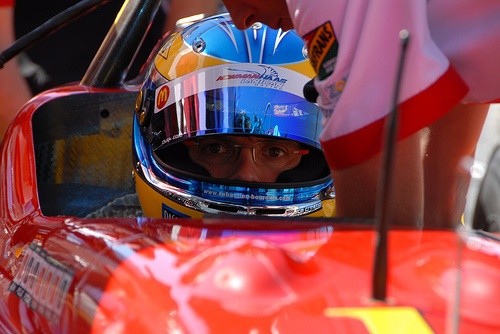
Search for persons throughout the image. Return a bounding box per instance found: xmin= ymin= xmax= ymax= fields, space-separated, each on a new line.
xmin=75 ymin=13 xmax=338 ymax=219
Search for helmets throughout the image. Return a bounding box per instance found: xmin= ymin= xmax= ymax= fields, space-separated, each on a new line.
xmin=127 ymin=12 xmax=336 ymax=219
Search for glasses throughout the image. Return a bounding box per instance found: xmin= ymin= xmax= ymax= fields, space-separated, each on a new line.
xmin=185 ymin=140 xmax=312 ymax=170
